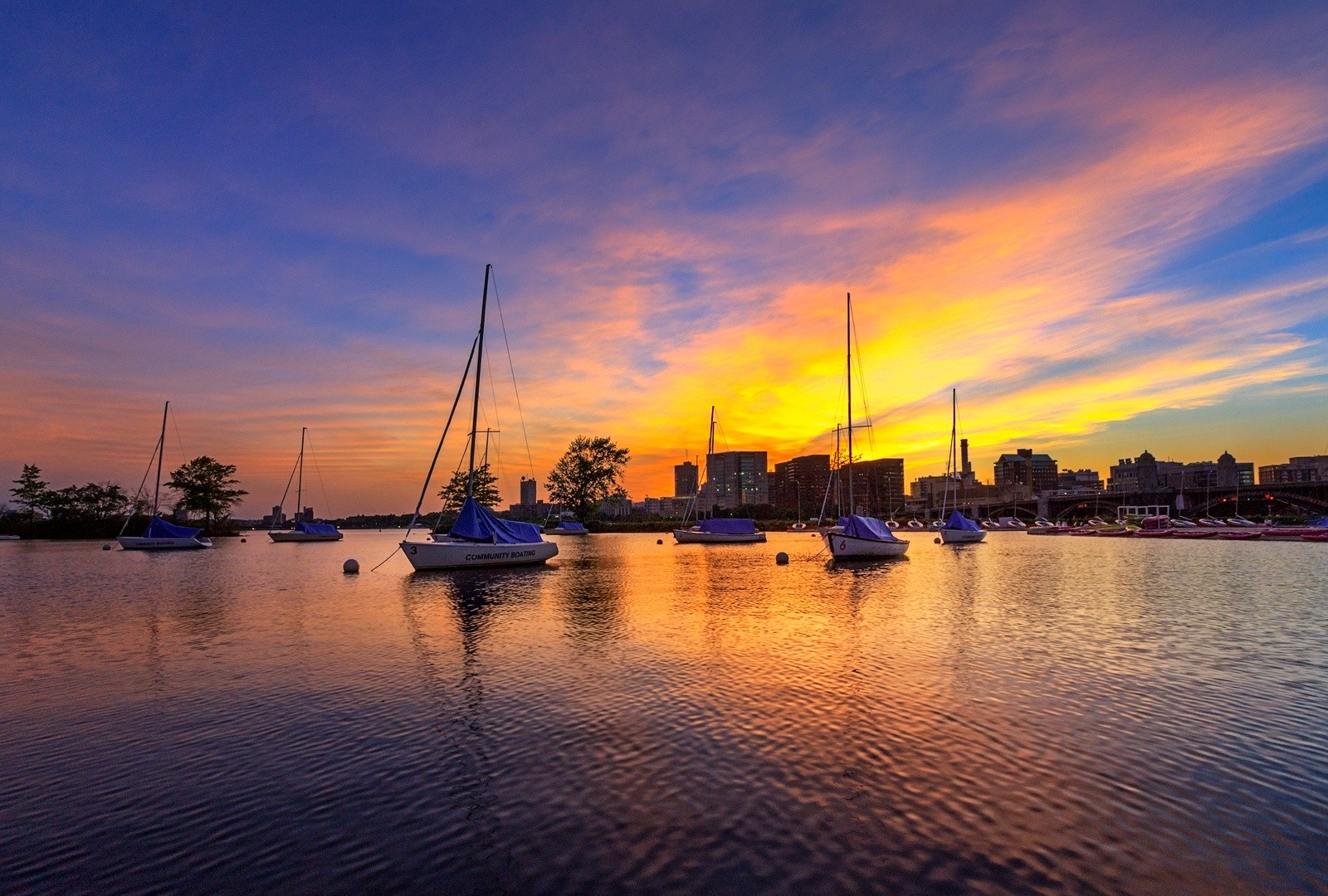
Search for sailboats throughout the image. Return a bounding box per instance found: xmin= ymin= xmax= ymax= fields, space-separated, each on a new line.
xmin=267 ymin=427 xmax=344 ymax=542
xmin=399 ymin=263 xmax=557 ymax=571
xmin=1034 ymin=479 xmax=1055 ymax=527
xmin=981 ymin=479 xmax=1001 ymax=529
xmin=540 ymin=482 xmax=589 ymax=535
xmin=117 ymin=401 xmax=213 ymax=551
xmin=786 ymin=480 xmax=818 ymax=532
xmin=1006 ymin=483 xmax=1027 ymax=528
xmin=814 ymin=290 xmax=909 ymax=561
xmin=674 ymin=406 xmax=767 ymax=544
xmin=939 ymin=389 xmax=987 ymax=544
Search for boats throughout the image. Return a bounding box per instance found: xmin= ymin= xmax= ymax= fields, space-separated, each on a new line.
xmin=908 ymin=478 xmax=923 ymax=530
xmin=1134 ymin=528 xmax=1177 ymax=537
xmin=1096 ymin=528 xmax=1134 ymax=537
xmin=884 ymin=477 xmax=900 ymax=529
xmin=1068 ymin=529 xmax=1096 ymax=536
xmin=931 ymin=481 xmax=946 ymax=528
xmin=1027 ymin=526 xmax=1060 ymax=535
xmin=1299 ymin=531 xmax=1328 ymax=542
xmin=1173 ymin=530 xmax=1218 ymax=539
xmin=0 ymin=534 xmax=21 ymax=540
xmin=1056 ymin=515 xmax=1268 ymax=528
xmin=1261 ymin=528 xmax=1328 ymax=536
xmin=1217 ymin=528 xmax=1263 ymax=541
